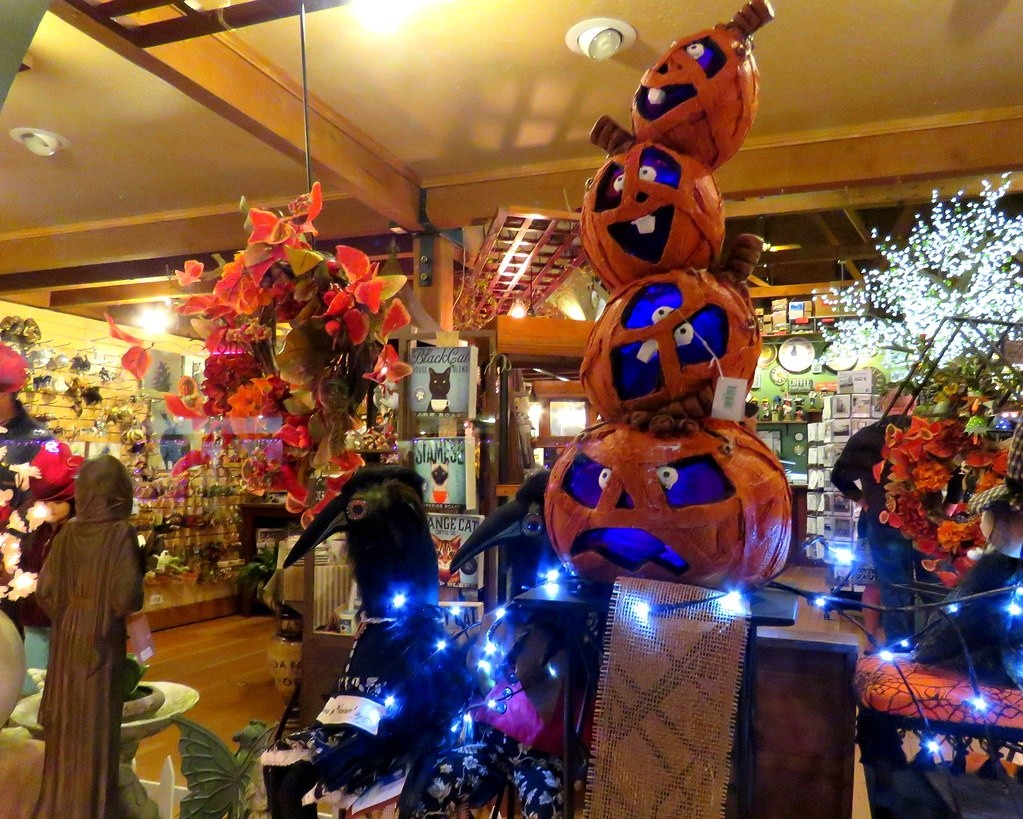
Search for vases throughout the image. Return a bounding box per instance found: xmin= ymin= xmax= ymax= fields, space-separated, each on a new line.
xmin=268 ymin=323 xmax=331 ymax=388
xmin=0 ymin=610 xmax=27 ymax=731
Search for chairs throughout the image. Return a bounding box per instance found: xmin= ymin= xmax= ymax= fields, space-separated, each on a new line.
xmin=814 ymin=579 xmax=1023 ymax=819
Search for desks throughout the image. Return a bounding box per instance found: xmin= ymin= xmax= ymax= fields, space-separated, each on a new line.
xmin=238 ymin=503 xmax=300 ymax=617
xmin=514 ymin=575 xmax=801 ymax=819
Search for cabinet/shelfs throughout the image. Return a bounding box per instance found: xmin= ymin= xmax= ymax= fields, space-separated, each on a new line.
xmin=789 ymin=485 xmax=808 ymax=567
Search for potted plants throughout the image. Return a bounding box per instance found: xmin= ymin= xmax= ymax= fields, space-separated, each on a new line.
xmin=231 ymin=541 xmax=303 ymax=730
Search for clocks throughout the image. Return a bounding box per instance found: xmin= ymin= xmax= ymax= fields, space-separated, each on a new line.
xmin=757 ymin=336 xmax=885 ymax=395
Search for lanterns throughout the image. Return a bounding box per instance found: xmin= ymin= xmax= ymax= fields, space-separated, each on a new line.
xmin=546 ymin=0 xmax=793 ymax=590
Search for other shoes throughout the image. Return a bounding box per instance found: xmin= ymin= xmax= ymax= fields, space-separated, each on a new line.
xmin=863 ymin=642 xmax=887 ymax=656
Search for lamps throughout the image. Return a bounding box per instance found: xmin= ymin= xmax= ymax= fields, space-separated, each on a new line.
xmin=10 ymin=127 xmax=70 ymax=157
xmin=564 ymin=18 xmax=637 ymax=62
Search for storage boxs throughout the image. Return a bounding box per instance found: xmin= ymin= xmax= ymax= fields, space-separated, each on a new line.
xmin=812 ymin=295 xmax=868 ymax=316
xmin=756 ymin=299 xmax=812 ymax=335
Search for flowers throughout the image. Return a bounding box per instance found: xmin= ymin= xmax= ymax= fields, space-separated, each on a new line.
xmin=872 ymin=332 xmax=1014 ymax=590
xmin=0 ymin=426 xmax=52 ymax=610
xmin=101 ymin=181 xmax=414 ymax=533
xmin=807 ymin=170 xmax=1023 ymax=383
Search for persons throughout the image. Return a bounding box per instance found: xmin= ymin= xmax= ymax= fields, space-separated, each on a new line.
xmin=809 ymin=387 xmax=827 ymax=410
xmin=32 ymin=454 xmax=144 ymax=819
xmin=0 ymin=342 xmax=84 ymax=645
xmin=750 ymin=395 xmax=805 ymax=421
xmin=829 ymin=386 xmax=956 ymax=642
